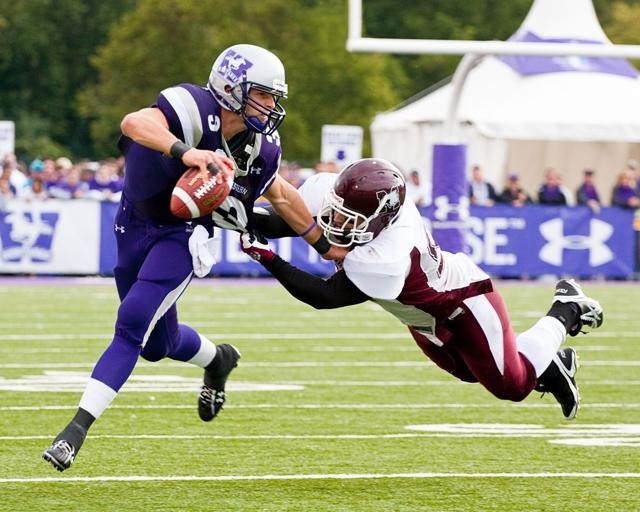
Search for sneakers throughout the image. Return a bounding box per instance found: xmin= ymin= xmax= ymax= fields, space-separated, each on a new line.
xmin=42 ymin=435 xmax=77 ymax=473
xmin=537 ymin=346 xmax=580 ymax=421
xmin=197 ymin=343 xmax=242 ymax=422
xmin=554 ymin=279 xmax=603 ymax=337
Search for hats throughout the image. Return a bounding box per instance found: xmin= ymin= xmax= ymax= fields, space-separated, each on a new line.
xmin=31 ymin=158 xmax=100 ymax=174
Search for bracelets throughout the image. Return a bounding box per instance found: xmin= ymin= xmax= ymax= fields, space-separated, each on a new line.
xmin=170 ymin=141 xmax=191 ymax=159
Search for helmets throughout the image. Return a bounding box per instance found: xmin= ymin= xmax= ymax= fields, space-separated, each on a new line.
xmin=316 ymin=157 xmax=407 ymax=251
xmin=206 ymin=43 xmax=289 ymax=135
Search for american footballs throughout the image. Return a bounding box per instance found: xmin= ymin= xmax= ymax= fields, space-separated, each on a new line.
xmin=170 ymin=158 xmax=236 ymax=218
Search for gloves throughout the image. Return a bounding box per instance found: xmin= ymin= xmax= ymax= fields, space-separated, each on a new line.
xmin=237 ymin=230 xmax=275 ymax=271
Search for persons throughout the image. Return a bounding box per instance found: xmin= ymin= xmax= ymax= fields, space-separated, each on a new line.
xmin=278 ymin=160 xmax=340 ymax=189
xmin=405 ymin=172 xmax=433 ymax=208
xmin=241 ymin=159 xmax=604 ymax=421
xmin=0 ymin=154 xmax=125 ymax=203
xmin=41 ymin=43 xmax=350 ymax=472
xmin=468 ymin=165 xmax=638 ymax=281
